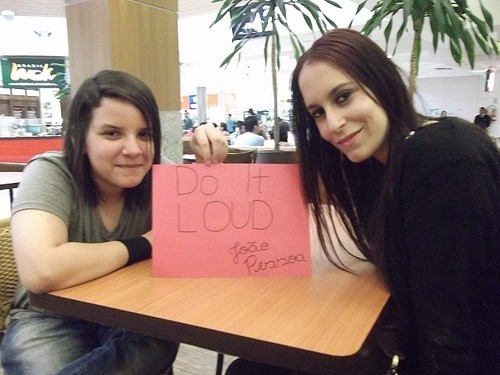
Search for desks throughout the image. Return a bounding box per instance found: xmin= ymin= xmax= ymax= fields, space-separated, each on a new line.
xmin=30 ymin=204 xmax=391 ymax=375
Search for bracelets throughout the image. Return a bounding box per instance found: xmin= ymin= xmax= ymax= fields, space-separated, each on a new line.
xmin=116 ymin=237 xmax=155 ymax=265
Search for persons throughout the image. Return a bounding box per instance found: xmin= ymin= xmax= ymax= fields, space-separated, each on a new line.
xmin=183 ymin=109 xmax=292 ymax=150
xmin=225 ymin=27 xmax=500 ymax=374
xmin=0 ymin=69 xmax=183 ymax=375
xmin=473 ymin=107 xmax=492 ymax=131
